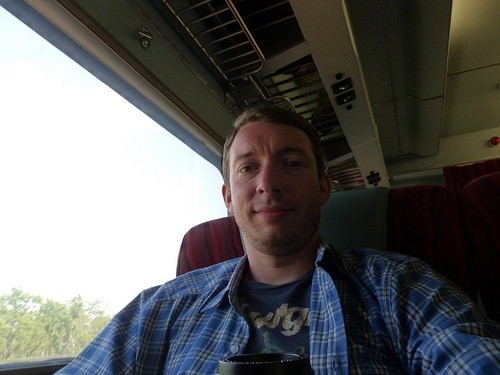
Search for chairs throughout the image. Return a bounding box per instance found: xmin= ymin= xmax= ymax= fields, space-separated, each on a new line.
xmin=459 ymin=172 xmax=500 ymax=229
xmin=177 ymin=184 xmax=500 ymax=329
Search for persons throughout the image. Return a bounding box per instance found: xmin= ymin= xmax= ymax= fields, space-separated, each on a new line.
xmin=51 ymin=105 xmax=500 ymax=375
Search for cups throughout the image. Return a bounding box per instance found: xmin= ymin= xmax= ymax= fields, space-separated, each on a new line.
xmin=214 ymin=352 xmax=313 ymax=375
